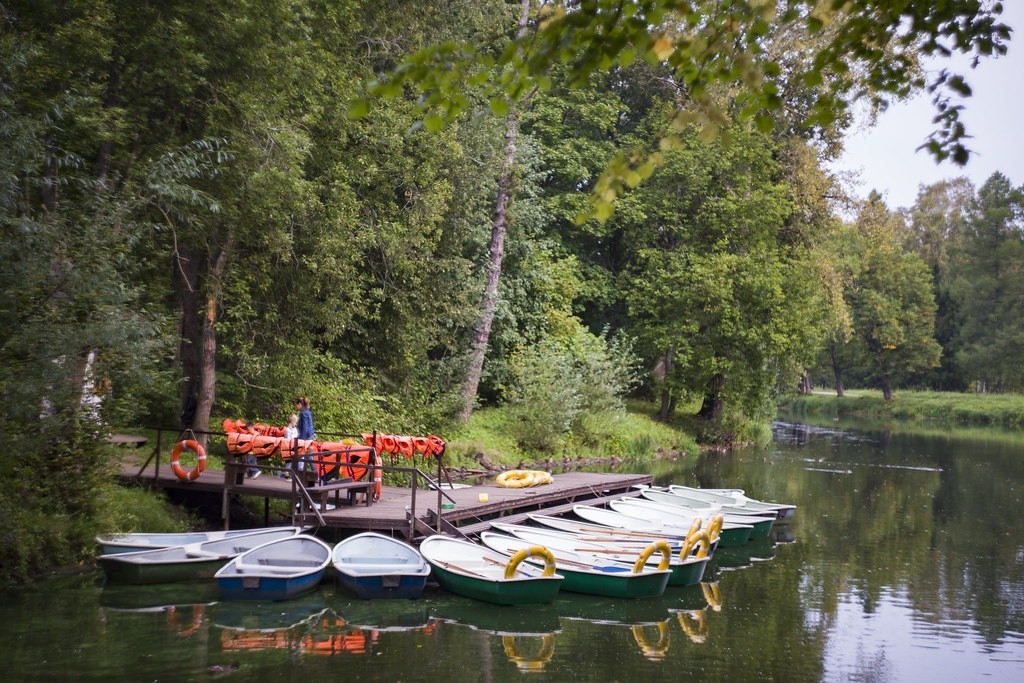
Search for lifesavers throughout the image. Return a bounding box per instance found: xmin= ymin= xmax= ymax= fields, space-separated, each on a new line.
xmin=501 ymin=633 xmax=558 ymax=671
xmin=373 ymin=455 xmax=382 ymax=502
xmin=503 ymin=544 xmax=557 ymax=580
xmin=677 ymin=529 xmax=711 ymax=561
xmin=170 ymin=439 xmax=207 ymax=481
xmin=685 ymin=516 xmax=702 ymax=543
xmin=701 ymin=582 xmax=724 ymax=607
xmin=677 ymin=611 xmax=710 ymax=639
xmin=706 ymin=515 xmax=723 ymax=541
xmin=632 ymin=540 xmax=672 ymax=573
xmin=632 ymin=621 xmax=674 ymax=655
xmin=495 ymin=468 xmax=551 ymax=489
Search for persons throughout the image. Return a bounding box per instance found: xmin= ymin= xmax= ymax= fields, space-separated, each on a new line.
xmin=278 ymin=396 xmax=320 ymax=482
xmin=243 ymin=421 xmax=261 ymax=479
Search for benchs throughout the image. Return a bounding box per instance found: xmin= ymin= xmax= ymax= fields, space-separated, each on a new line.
xmin=348 ymin=564 xmax=419 ymax=573
xmin=474 ymin=567 xmax=503 ymax=574
xmin=299 ymin=478 xmax=376 ymax=512
xmin=728 ymin=504 xmax=745 ymax=507
xmin=235 ymin=563 xmax=317 ymax=574
xmin=185 ymin=549 xmax=228 ymax=560
xmin=109 ymin=433 xmax=149 ymax=450
xmin=698 ymin=508 xmax=722 ymax=513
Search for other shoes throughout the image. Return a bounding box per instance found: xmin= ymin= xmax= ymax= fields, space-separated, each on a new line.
xmin=287 ymin=478 xmax=292 ymax=482
xmin=284 ymin=474 xmax=291 ymax=481
xmin=252 ymin=471 xmax=262 ymax=480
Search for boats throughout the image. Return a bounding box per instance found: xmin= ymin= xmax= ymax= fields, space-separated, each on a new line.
xmin=212 ymin=534 xmax=332 ymax=601
xmin=211 ymin=527 xmax=795 ymax=638
xmin=609 ymin=500 xmax=753 ymax=548
xmin=95 ymin=522 xmax=293 ymax=554
xmin=633 ymin=483 xmax=745 ymax=495
xmin=490 ymin=522 xmax=710 ymax=586
xmin=642 ymin=489 xmax=777 ymax=520
xmin=480 ymin=531 xmax=674 ymax=600
xmin=572 ymin=504 xmax=723 ymax=560
xmin=332 ymin=531 xmax=432 ymax=600
xmin=97 ymin=527 xmax=301 ymax=584
xmin=526 ymin=513 xmax=698 ymax=557
xmin=100 ymin=580 xmax=218 ymax=613
xmin=621 ymin=496 xmax=774 ymax=539
xmin=669 ymin=485 xmax=797 ymax=525
xmin=418 ymin=535 xmax=565 ymax=605
